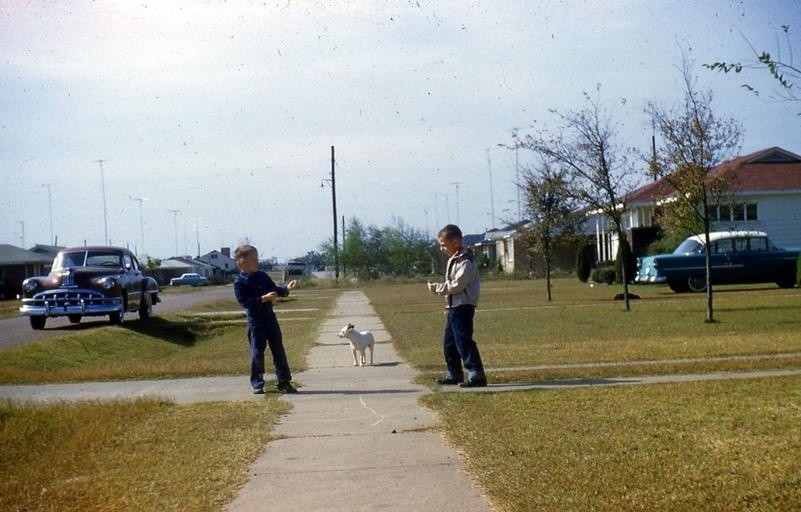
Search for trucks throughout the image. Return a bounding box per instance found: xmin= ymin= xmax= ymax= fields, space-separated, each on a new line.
xmin=281 ymin=256 xmax=311 ymax=282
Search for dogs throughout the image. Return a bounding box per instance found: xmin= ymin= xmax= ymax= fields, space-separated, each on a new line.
xmin=337 ymin=323 xmax=375 ymax=367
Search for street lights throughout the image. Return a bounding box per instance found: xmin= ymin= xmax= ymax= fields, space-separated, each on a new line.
xmin=320 ymin=179 xmax=340 ymax=280
xmin=16 ymin=220 xmax=25 ymax=249
xmin=168 ymin=207 xmax=183 ymax=256
xmin=92 ymin=159 xmax=109 ymax=245
xmin=41 ymin=183 xmax=54 ymax=247
xmin=133 ymin=198 xmax=145 ymax=257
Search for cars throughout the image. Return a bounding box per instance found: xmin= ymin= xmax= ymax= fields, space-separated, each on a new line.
xmin=16 ymin=246 xmax=162 ymax=330
xmin=170 ymin=272 xmax=211 ymax=287
xmin=369 ymin=260 xmax=433 ymax=280
xmin=635 ymin=230 xmax=801 ymax=293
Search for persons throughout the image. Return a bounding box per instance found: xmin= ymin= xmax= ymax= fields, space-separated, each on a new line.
xmin=233 ymin=245 xmax=302 ymax=393
xmin=427 ymin=225 xmax=487 ymax=387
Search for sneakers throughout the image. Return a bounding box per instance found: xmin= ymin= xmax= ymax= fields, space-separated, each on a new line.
xmin=457 ymin=378 xmax=487 ymax=388
xmin=436 ymin=377 xmax=464 ymax=385
xmin=276 ymin=382 xmax=297 ymax=393
xmin=253 ymin=387 xmax=263 ymax=394
